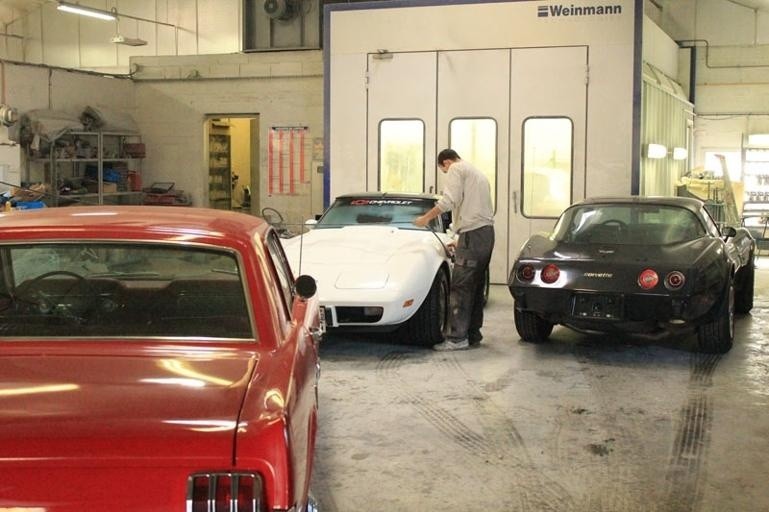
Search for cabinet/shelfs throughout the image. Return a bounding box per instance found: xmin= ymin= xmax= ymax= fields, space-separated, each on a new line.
xmin=208 ymin=133 xmax=232 ymax=210
xmin=24 ymin=129 xmax=143 ymax=206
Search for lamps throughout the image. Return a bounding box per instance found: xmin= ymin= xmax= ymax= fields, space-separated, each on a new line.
xmin=56 ymin=0 xmax=148 ymax=47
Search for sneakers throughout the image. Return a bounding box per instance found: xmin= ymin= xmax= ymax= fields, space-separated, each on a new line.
xmin=433 ymin=338 xmax=469 ymax=351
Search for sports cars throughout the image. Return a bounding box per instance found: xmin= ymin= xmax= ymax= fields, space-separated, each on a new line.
xmin=271 ymin=187 xmax=492 ymax=351
xmin=500 ymin=193 xmax=760 ymax=362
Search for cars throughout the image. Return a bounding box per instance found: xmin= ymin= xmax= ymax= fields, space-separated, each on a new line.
xmin=1 ymin=200 xmax=336 ymax=512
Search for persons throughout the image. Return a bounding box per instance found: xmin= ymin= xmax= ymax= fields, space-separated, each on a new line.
xmin=414 ymin=150 xmax=495 ymax=350
